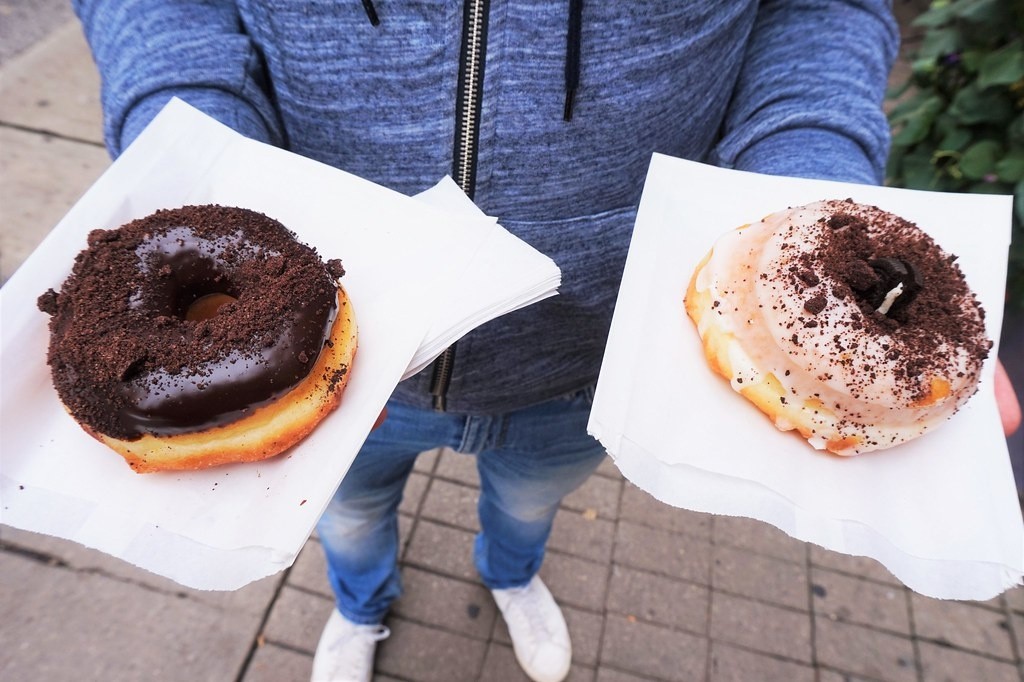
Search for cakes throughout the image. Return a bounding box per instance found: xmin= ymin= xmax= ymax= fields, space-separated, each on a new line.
xmin=684 ymin=202 xmax=994 ymax=454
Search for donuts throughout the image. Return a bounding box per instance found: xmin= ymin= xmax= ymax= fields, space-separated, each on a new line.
xmin=36 ymin=204 xmax=356 ymax=474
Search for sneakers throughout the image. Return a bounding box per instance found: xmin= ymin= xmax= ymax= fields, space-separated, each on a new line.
xmin=309 ymin=605 xmax=392 ymax=682
xmin=491 ymin=574 xmax=572 ymax=682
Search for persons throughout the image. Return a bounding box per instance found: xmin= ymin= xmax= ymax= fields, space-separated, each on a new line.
xmin=65 ymin=0 xmax=1024 ymax=682
